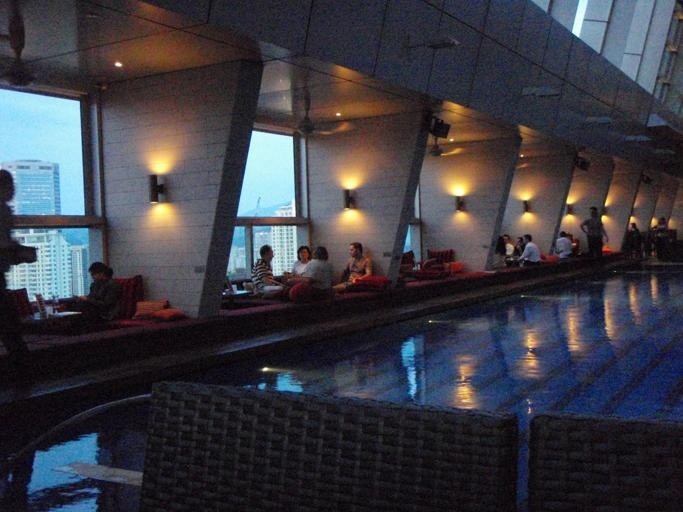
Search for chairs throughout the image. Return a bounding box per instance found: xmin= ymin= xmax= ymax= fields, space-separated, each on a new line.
xmin=525 ymin=410 xmax=681 ymax=512
xmin=0 ymin=376 xmax=526 ymax=512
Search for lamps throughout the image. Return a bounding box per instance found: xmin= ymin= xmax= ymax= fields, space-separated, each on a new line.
xmin=342 ymin=188 xmax=352 ymax=212
xmin=602 ymin=204 xmax=608 ymax=217
xmin=410 ymin=34 xmax=462 ymax=57
xmin=521 ymin=198 xmax=531 ymax=217
xmin=564 ymin=203 xmax=574 ymax=220
xmin=523 ymin=83 xmax=681 ymax=168
xmin=146 ymin=172 xmax=158 ymax=205
xmin=453 ymin=194 xmax=465 ymax=215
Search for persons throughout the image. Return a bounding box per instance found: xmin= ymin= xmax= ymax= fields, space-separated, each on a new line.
xmin=492 ymin=235 xmax=508 ymax=268
xmin=0 ymin=169 xmax=38 ymax=338
xmin=502 ymin=233 xmax=514 ymax=257
xmin=330 ymin=242 xmax=373 ymax=292
xmin=650 ymin=217 xmax=667 ymax=257
xmin=281 ymin=246 xmax=311 ymax=296
xmin=511 ymin=236 xmax=525 ymax=266
xmin=249 ymin=244 xmax=290 ymax=295
xmin=556 ymin=230 xmax=573 ymax=259
xmin=516 ymin=232 xmax=541 ymax=266
xmin=69 ymin=259 xmax=123 ymax=336
xmin=630 ymin=223 xmax=642 ymax=259
xmin=579 ymin=206 xmax=610 ymax=258
xmin=287 ymin=247 xmax=333 ymax=302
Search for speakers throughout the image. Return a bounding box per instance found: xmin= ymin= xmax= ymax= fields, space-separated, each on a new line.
xmin=433 ymin=121 xmax=451 ymax=139
xmin=581 ymin=161 xmax=591 ymax=170
xmin=650 ymin=178 xmax=657 ymax=186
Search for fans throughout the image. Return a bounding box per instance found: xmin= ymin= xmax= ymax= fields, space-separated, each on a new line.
xmin=425 ymin=131 xmax=466 ymax=158
xmin=271 ymin=89 xmax=347 ymax=139
xmin=0 ymin=12 xmax=50 ymax=92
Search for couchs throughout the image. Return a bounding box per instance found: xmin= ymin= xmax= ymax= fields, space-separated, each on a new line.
xmin=5 ymin=272 xmax=198 ymax=358
xmin=399 ymin=251 xmax=488 ymax=299
xmin=223 ymin=262 xmax=391 ymax=333
xmin=494 ymin=240 xmax=609 ymax=285
